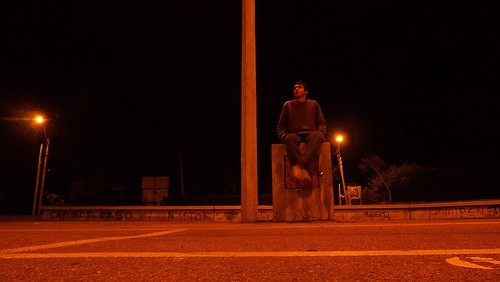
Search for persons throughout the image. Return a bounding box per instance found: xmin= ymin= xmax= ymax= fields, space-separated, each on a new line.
xmin=278 ymin=80 xmax=326 ymax=182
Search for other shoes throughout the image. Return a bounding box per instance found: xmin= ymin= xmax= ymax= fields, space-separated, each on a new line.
xmin=292 ymin=165 xmax=302 ymax=179
xmin=300 ymin=169 xmax=311 ymax=183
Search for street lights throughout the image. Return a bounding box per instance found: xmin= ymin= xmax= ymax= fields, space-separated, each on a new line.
xmin=333 ymin=130 xmax=350 ymax=207
xmin=32 ymin=112 xmax=50 ymax=216
